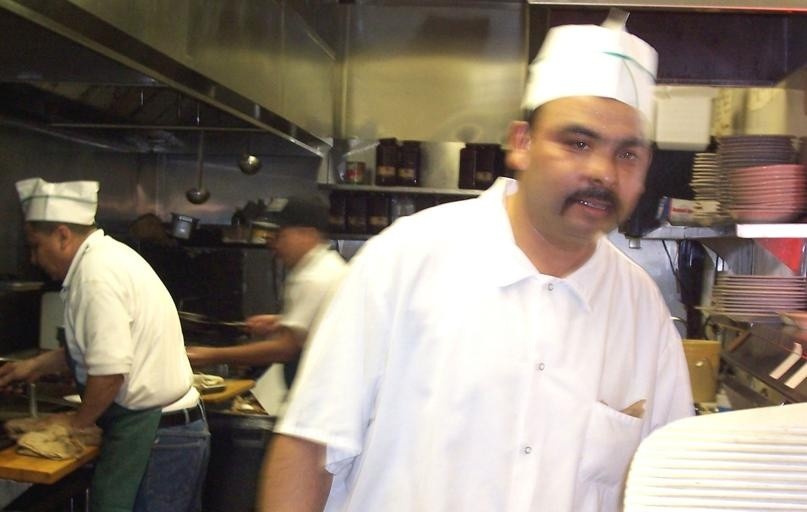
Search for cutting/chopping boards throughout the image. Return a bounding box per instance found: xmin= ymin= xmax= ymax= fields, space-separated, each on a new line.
xmin=199 ymin=376 xmax=256 ymax=407
xmin=0 ymin=442 xmax=103 ymax=485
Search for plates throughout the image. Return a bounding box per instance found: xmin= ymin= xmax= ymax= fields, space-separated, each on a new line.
xmin=688 ymin=152 xmax=721 ymax=227
xmin=694 ymin=273 xmax=807 ymax=331
xmin=727 ymin=163 xmax=807 ymax=224
xmin=714 ymin=133 xmax=798 ymax=223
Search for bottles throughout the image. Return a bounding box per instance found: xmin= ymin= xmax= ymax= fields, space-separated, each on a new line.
xmin=457 ymin=143 xmax=513 ymax=191
xmin=327 ymin=190 xmax=415 ymax=235
xmin=344 ymin=159 xmax=366 ymax=184
xmin=374 ymin=137 xmax=422 ymax=188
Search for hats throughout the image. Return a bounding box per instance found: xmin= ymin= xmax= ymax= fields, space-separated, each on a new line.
xmin=251 ymin=196 xmax=331 ymax=229
xmin=14 ymin=178 xmax=99 ymax=226
xmin=522 ymin=26 xmax=659 ymax=123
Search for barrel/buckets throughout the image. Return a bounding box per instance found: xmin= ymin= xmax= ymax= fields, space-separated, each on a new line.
xmin=205 ymin=406 xmax=282 ymax=512
xmin=682 ymin=338 xmax=722 ymax=406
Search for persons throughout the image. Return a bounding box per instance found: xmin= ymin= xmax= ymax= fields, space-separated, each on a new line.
xmin=256 ymin=24 xmax=695 ymax=512
xmin=184 ymin=196 xmax=347 ymax=421
xmin=0 ymin=176 xmax=211 ymax=512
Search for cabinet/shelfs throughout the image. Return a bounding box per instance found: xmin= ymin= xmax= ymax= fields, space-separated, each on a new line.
xmin=317 ymin=182 xmax=486 ymax=241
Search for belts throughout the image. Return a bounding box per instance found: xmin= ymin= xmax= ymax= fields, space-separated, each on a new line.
xmin=158 ymin=397 xmax=206 ymax=429
